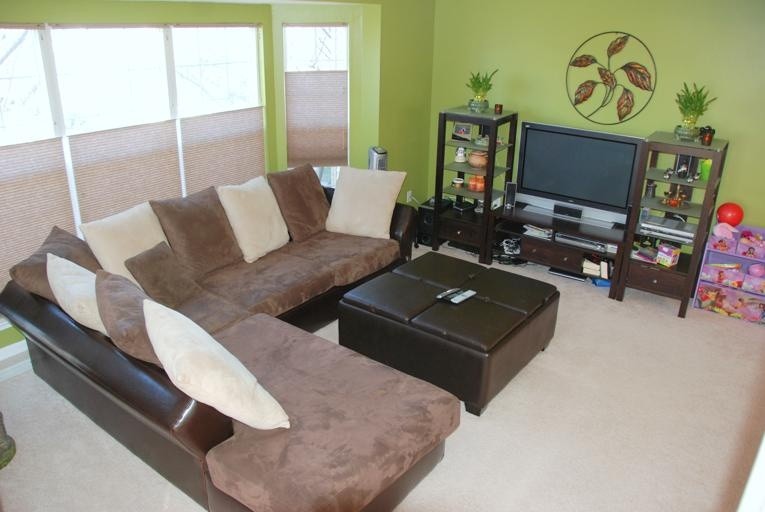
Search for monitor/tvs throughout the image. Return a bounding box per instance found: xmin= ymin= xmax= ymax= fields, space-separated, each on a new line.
xmin=514 ymin=120 xmax=646 ymax=229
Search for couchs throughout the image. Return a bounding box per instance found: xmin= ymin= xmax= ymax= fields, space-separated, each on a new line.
xmin=0 ymin=183 xmax=473 ymax=512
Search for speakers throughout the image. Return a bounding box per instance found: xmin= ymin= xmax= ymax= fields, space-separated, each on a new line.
xmin=504 ymin=182 xmax=517 ymax=209
xmin=418 ymin=197 xmax=453 ymax=247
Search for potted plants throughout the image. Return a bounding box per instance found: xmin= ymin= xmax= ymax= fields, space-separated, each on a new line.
xmin=463 ymin=65 xmax=501 ymax=115
xmin=671 ymin=78 xmax=721 ymax=145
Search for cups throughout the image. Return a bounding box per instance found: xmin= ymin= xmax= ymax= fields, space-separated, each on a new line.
xmin=646 ymin=183 xmax=657 ymax=200
xmin=495 ymin=104 xmax=503 ymax=114
xmin=451 ymin=178 xmax=464 ymax=189
xmin=702 ymin=129 xmax=716 ymax=147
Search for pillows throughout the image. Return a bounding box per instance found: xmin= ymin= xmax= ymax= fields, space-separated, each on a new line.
xmin=123 ymin=240 xmax=203 ymax=311
xmin=145 ymin=184 xmax=246 ymax=282
xmin=38 ymin=244 xmax=111 ymax=341
xmin=85 ymin=263 xmax=161 ymax=368
xmin=325 ymin=164 xmax=407 ymax=241
xmin=212 ymin=173 xmax=290 ymax=264
xmin=8 ymin=221 xmax=107 ymax=299
xmin=138 ymin=291 xmax=296 ymax=437
xmin=74 ymin=198 xmax=172 ymax=289
xmin=264 ymin=162 xmax=336 ymax=243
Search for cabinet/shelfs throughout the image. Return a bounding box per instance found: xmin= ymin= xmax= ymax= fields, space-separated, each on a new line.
xmin=614 ymin=125 xmax=731 ymax=318
xmin=485 ymin=198 xmax=626 ymax=304
xmin=691 ymin=215 xmax=765 ymax=325
xmin=427 ymin=98 xmax=519 ymax=267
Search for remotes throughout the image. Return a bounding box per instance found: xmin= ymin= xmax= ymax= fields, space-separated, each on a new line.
xmin=451 ymin=290 xmax=476 ymax=304
xmin=443 ymin=290 xmax=466 ymax=301
xmin=436 ymin=288 xmax=461 ymax=299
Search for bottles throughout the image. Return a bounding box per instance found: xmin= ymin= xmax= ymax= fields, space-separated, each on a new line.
xmin=455 ymin=147 xmax=466 ymax=163
xmin=642 ymin=206 xmax=649 ymax=221
